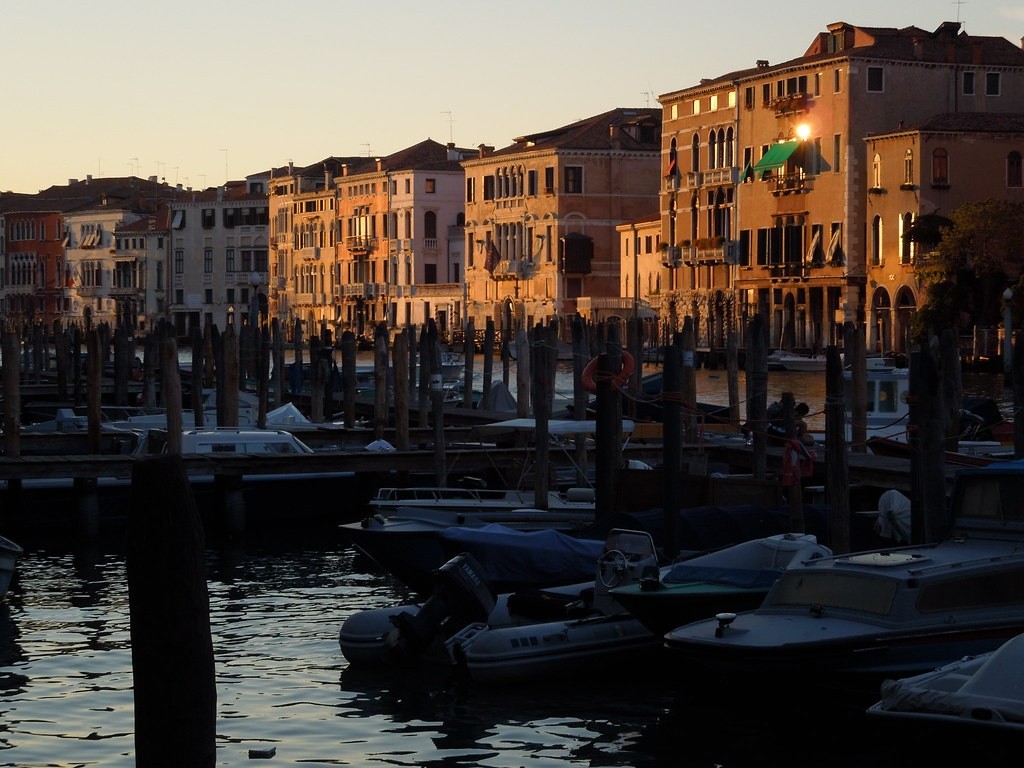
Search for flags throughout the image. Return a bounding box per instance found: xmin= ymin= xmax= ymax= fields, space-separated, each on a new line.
xmin=483 ymin=240 xmax=502 ymax=274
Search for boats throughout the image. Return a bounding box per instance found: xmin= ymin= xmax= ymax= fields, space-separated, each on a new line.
xmin=767 ymin=350 xmax=800 ymax=371
xmin=776 ymin=354 xmax=846 ymax=372
xmin=0 ymin=351 xmax=740 ymax=540
xmin=446 ymin=527 xmax=835 ymax=682
xmin=661 ymin=457 xmax=1024 ymax=718
xmin=864 ymin=632 xmax=1024 ymax=768
xmin=840 ymin=358 xmax=911 ymax=455
xmin=338 ymin=431 xmax=1024 ymax=610
xmin=509 ymin=341 xmax=575 ymax=361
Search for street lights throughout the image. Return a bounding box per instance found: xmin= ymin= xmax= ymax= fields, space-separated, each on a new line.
xmin=250 ymin=272 xmax=262 ymax=340
xmin=1002 ymin=288 xmax=1014 ymax=388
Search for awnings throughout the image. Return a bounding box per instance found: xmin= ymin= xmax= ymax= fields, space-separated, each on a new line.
xmin=753 ymin=138 xmax=804 ymax=171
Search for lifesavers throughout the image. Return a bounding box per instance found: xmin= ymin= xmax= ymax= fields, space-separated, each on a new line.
xmin=580 ymin=347 xmax=637 ymax=393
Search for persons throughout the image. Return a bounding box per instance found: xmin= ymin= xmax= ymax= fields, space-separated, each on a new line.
xmin=768 ymin=402 xmax=814 ymax=447
